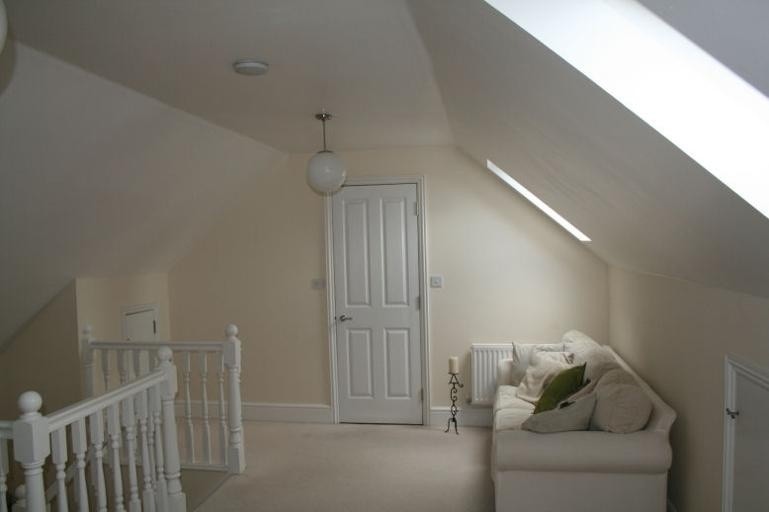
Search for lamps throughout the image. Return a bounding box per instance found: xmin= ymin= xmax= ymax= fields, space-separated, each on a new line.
xmin=305 ymin=113 xmax=346 ymax=193
xmin=233 ymin=58 xmax=269 ymax=75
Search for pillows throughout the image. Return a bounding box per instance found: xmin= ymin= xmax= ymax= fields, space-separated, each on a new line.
xmin=516 ymin=330 xmax=654 ymax=433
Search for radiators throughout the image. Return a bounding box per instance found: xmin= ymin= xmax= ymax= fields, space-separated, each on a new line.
xmin=471 ymin=343 xmax=513 ymax=404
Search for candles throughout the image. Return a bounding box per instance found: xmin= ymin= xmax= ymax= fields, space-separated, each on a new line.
xmin=450 ymin=356 xmax=458 ymax=372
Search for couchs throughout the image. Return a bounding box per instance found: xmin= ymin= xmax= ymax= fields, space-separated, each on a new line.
xmin=488 ymin=342 xmax=676 ymax=512
